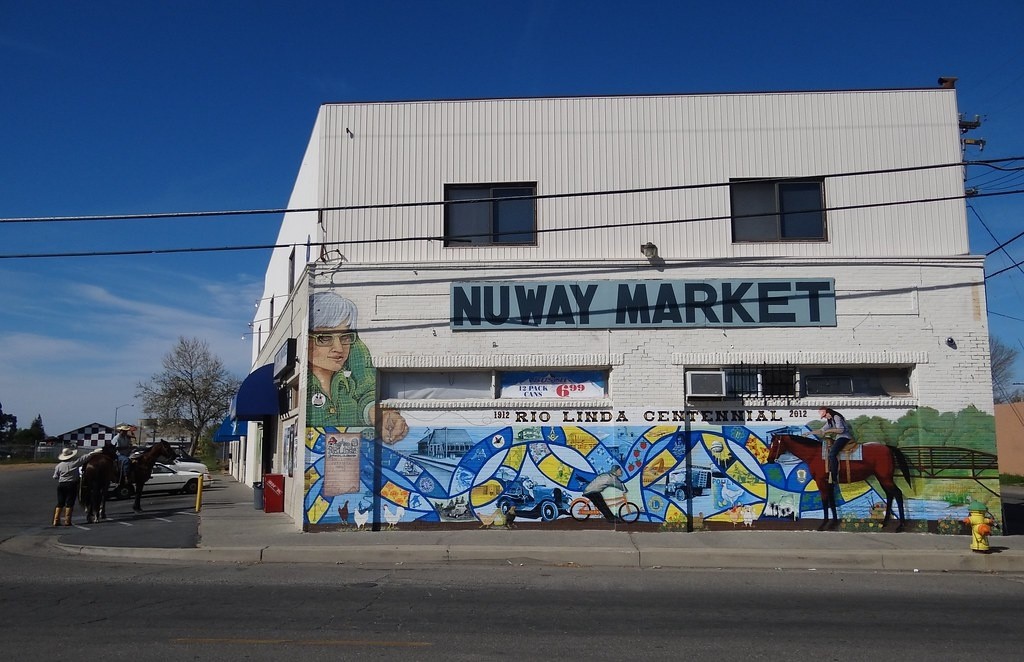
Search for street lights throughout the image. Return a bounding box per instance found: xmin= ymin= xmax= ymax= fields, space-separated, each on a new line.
xmin=114 ymin=404 xmax=134 ymax=436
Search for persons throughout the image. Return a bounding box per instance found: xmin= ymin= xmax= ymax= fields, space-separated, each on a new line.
xmin=111 ymin=426 xmax=133 ymax=483
xmin=52 ymin=447 xmax=95 ymax=526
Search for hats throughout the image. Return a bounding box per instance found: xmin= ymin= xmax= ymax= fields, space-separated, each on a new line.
xmin=58 ymin=448 xmax=78 ymax=460
xmin=116 ymin=426 xmax=130 ymax=430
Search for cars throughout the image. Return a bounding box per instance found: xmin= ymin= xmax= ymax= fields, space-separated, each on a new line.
xmin=108 ymin=461 xmax=213 ymax=500
xmin=130 ymin=451 xmax=210 ymax=474
xmin=0 ymin=450 xmax=14 ymax=460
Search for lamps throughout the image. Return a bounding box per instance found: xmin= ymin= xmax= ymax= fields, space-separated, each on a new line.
xmin=248 ymin=315 xmax=279 ymax=329
xmin=254 ymin=294 xmax=291 ymax=309
xmin=640 ymin=241 xmax=658 ymax=260
xmin=241 ymin=330 xmax=271 ymax=341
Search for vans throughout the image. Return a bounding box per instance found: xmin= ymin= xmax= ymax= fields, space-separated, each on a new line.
xmin=172 ymin=447 xmax=201 ymax=463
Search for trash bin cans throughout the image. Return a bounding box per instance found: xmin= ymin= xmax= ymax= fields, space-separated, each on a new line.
xmin=252 ymin=481 xmax=264 ymax=510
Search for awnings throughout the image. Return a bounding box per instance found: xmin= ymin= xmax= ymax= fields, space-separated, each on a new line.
xmin=213 ymin=429 xmax=240 ymax=442
xmin=230 ymin=363 xmax=281 ymax=422
xmin=218 ymin=416 xmax=246 ymax=437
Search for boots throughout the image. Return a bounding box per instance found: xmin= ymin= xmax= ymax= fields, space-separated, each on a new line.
xmin=65 ymin=507 xmax=72 ymax=525
xmin=53 ymin=507 xmax=62 ymax=526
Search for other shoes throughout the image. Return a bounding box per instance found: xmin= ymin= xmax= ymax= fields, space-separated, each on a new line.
xmin=125 ymin=480 xmax=131 ymax=484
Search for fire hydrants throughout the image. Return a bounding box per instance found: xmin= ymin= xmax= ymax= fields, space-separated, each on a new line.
xmin=962 ymin=501 xmax=996 ymax=553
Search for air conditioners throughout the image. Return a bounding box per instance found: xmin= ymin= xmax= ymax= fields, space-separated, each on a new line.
xmin=685 ymin=371 xmax=726 ymax=397
